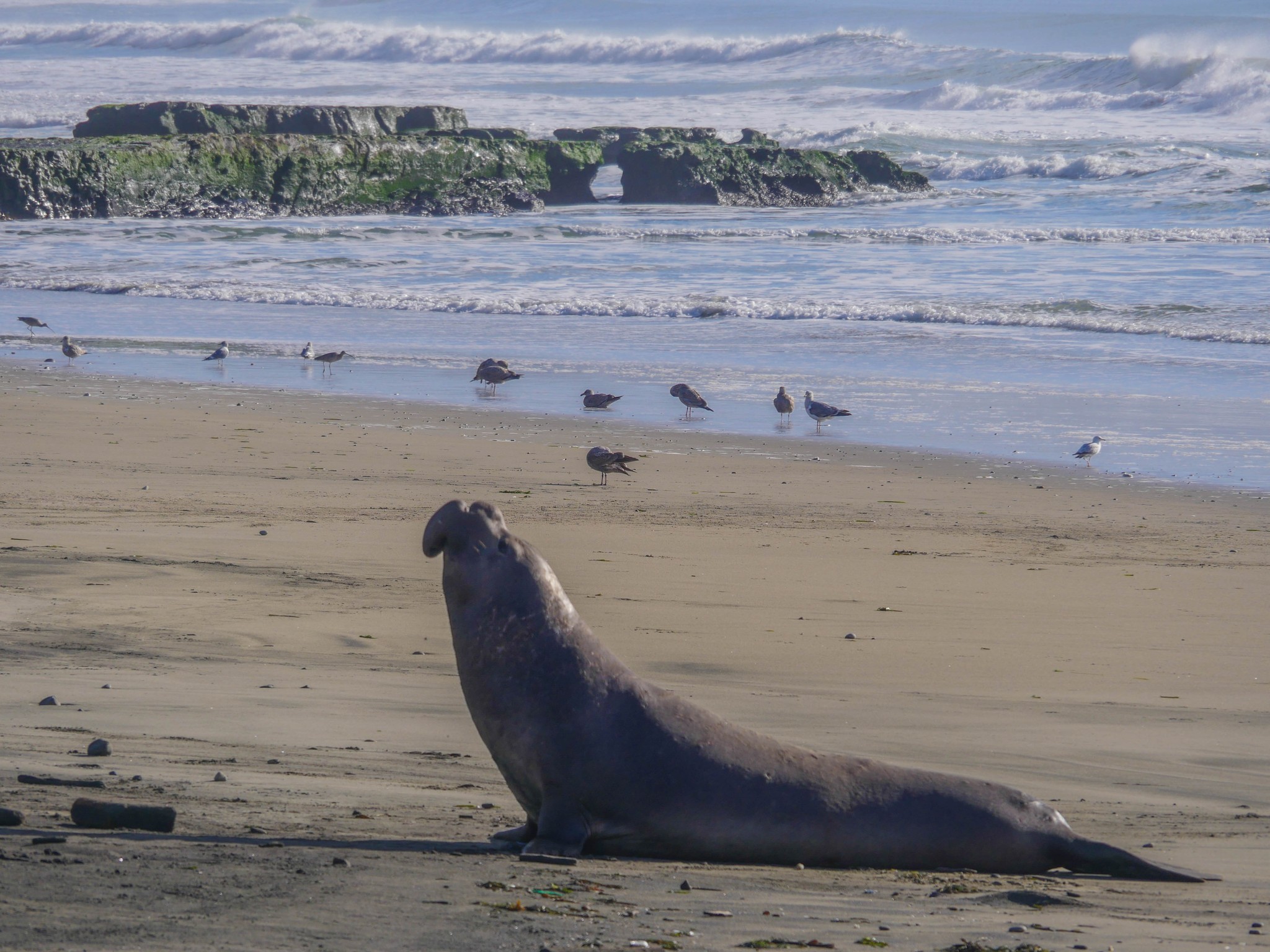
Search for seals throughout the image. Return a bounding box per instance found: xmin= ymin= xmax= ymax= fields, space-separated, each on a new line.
xmin=420 ymin=496 xmax=1223 ymax=882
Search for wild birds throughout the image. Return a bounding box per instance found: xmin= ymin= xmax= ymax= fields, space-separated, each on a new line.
xmin=580 ymin=389 xmax=624 ymax=407
xmin=1072 ymin=436 xmax=1106 ymax=468
xmin=470 ymin=358 xmax=525 ymax=393
xmin=804 ymin=390 xmax=853 ymax=432
xmin=586 ymin=446 xmax=640 ymax=486
xmin=300 ymin=342 xmax=314 ymax=364
xmin=60 ymin=336 xmax=93 ymax=361
xmin=670 ymin=383 xmax=714 ymax=415
xmin=201 ymin=341 xmax=229 ymax=364
xmin=17 ymin=316 xmax=56 ymax=337
xmin=773 ymin=386 xmax=795 ymax=422
xmin=313 ymin=351 xmax=356 ymax=375
